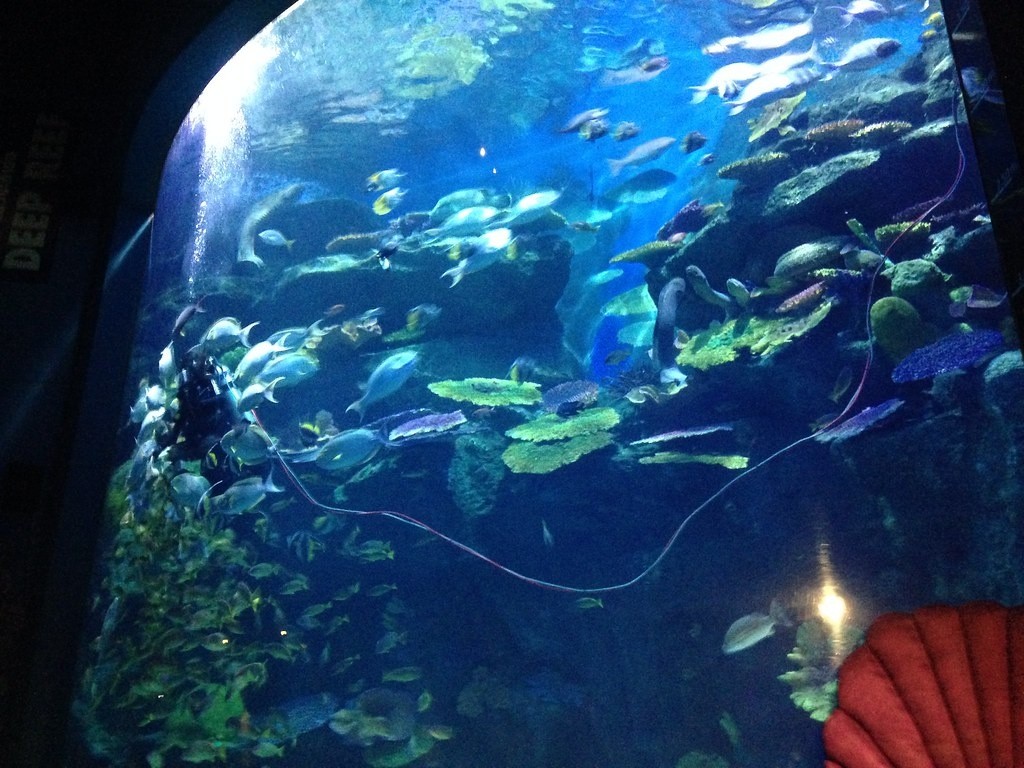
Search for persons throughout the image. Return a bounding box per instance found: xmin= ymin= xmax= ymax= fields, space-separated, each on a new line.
xmin=157 ymin=350 xmax=272 ymax=533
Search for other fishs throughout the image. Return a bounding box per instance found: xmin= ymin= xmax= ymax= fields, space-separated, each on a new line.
xmin=556 ymin=0 xmax=719 ymax=224
xmin=125 ymin=290 xmax=423 ymax=517
xmin=827 ymin=366 xmax=853 ymax=404
xmin=258 ymin=229 xmax=296 ymax=252
xmin=507 ymin=261 xmax=658 ymax=419
xmin=574 ymin=597 xmax=604 ymax=609
xmin=702 ymin=0 xmax=814 ymax=56
xmin=818 ymin=37 xmax=902 ymax=82
xmin=70 ymin=510 xmax=458 ymax=768
xmin=684 ymin=221 xmax=862 ymax=325
xmin=824 ymin=0 xmax=889 ymax=31
xmin=364 ymin=168 xmax=567 ymax=290
xmin=234 ymin=183 xmax=305 ymax=271
xmin=721 ymin=595 xmax=794 ymax=655
xmin=687 ymin=61 xmax=822 ymax=117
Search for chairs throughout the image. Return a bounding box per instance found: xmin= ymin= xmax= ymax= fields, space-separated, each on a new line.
xmin=823 ymin=603 xmax=1024 ymax=768
